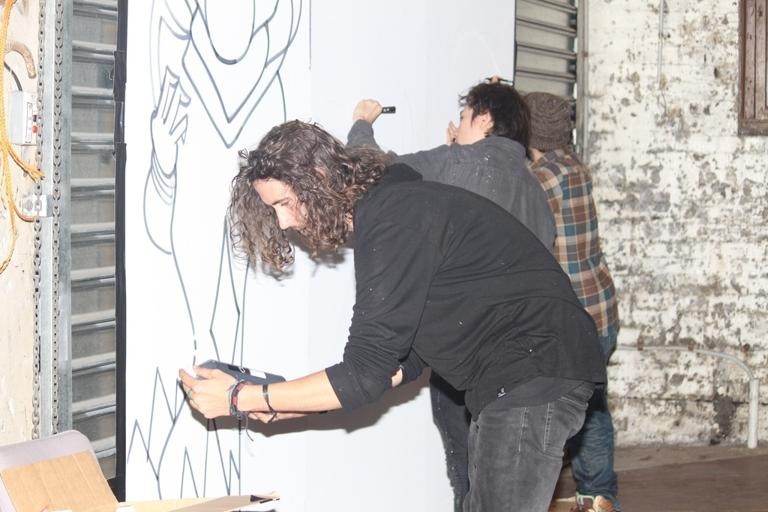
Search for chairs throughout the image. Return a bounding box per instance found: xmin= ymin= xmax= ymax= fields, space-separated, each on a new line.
xmin=0 ymin=427 xmax=122 ymax=510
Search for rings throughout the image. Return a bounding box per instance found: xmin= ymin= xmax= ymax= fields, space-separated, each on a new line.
xmin=185 ymin=390 xmax=192 ymax=401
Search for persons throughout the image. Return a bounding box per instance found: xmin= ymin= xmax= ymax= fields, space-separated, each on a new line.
xmin=524 ymin=90 xmax=621 ymax=512
xmin=349 ymin=76 xmax=560 ymax=512
xmin=178 ymin=119 xmax=598 ymax=512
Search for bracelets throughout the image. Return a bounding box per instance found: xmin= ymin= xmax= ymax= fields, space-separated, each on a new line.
xmin=261 ymin=383 xmax=274 ymax=414
xmin=227 ymin=378 xmax=249 ymax=421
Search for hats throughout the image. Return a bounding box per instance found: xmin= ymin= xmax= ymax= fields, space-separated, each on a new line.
xmin=523 ymin=90 xmax=575 ymax=154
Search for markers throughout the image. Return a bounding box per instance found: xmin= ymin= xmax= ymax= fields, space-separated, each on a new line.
xmin=380 ymin=107 xmax=396 ymax=114
